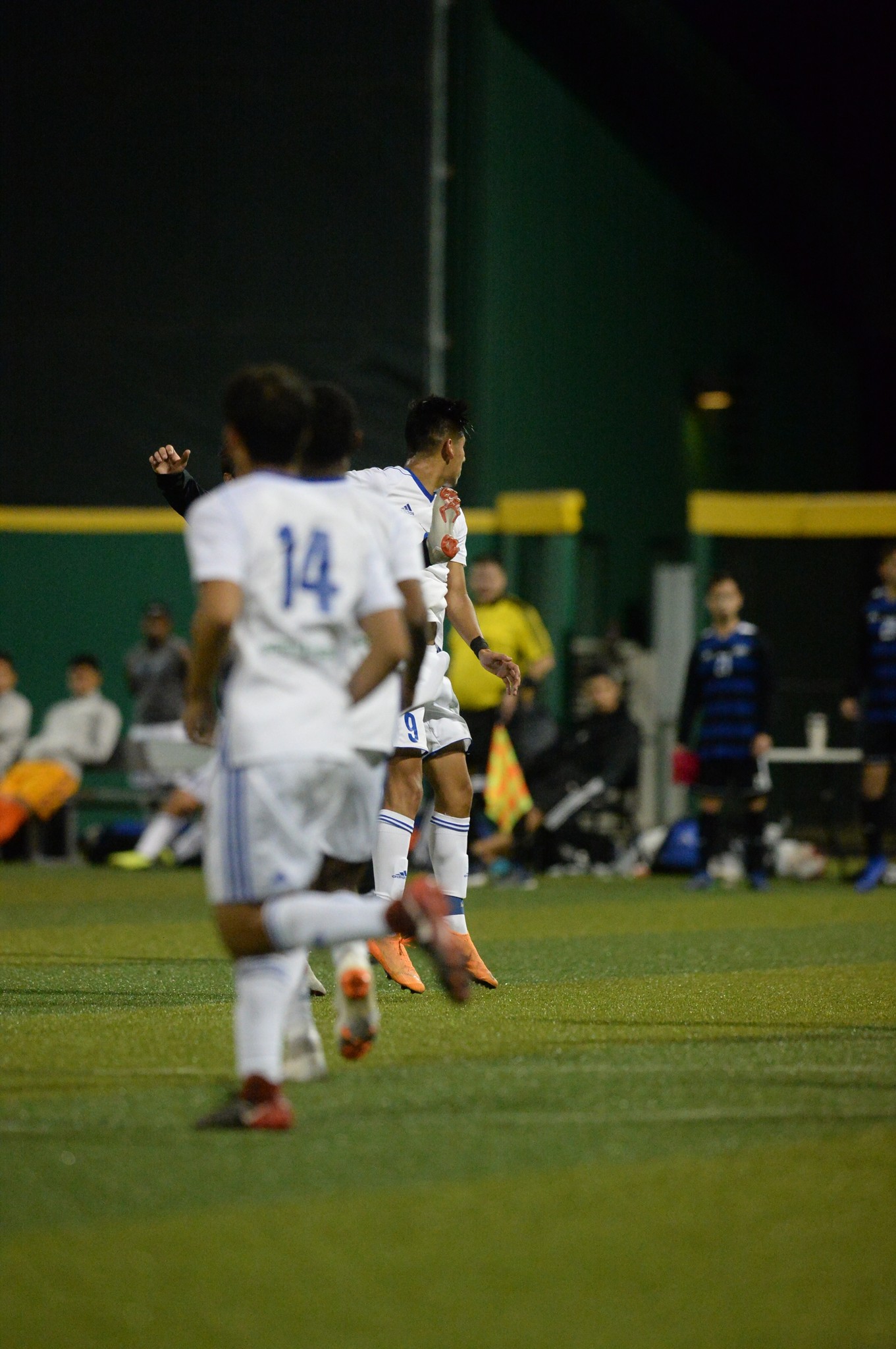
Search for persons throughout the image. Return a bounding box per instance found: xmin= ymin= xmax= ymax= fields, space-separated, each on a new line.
xmin=341 ymin=393 xmax=522 ymax=997
xmin=670 ymin=571 xmax=774 ymax=892
xmin=148 ymin=445 xmax=461 ymax=998
xmin=0 ymin=552 xmax=654 ymax=870
xmin=837 ymin=549 xmax=896 ymax=892
xmin=172 ymin=362 xmax=471 ymax=1133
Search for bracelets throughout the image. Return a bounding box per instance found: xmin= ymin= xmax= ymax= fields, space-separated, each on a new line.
xmin=469 ymin=635 xmax=489 ymax=659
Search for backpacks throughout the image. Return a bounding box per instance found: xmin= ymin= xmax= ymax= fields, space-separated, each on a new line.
xmin=651 ymin=823 xmax=707 ymax=881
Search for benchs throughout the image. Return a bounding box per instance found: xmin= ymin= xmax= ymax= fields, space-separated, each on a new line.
xmin=71 ymin=741 xmax=149 ymax=816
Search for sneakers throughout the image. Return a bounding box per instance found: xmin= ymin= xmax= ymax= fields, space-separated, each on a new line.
xmin=399 ymin=872 xmax=472 ymax=1004
xmin=335 ymin=956 xmax=379 ymax=1061
xmin=305 ymin=960 xmax=327 ymax=997
xmin=366 ymin=931 xmax=426 ymax=994
xmin=417 ymin=919 xmax=498 ymax=991
xmin=195 ymin=1077 xmax=292 ymax=1133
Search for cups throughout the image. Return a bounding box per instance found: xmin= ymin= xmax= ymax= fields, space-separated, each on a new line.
xmin=805 ymin=712 xmax=829 ymax=748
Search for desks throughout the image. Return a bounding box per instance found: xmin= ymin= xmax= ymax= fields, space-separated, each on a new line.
xmin=767 ymin=748 xmax=865 ymax=861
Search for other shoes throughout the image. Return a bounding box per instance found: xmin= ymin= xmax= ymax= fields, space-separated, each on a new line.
xmin=749 ymin=874 xmax=766 ymax=892
xmin=109 ymin=850 xmax=173 ymax=871
